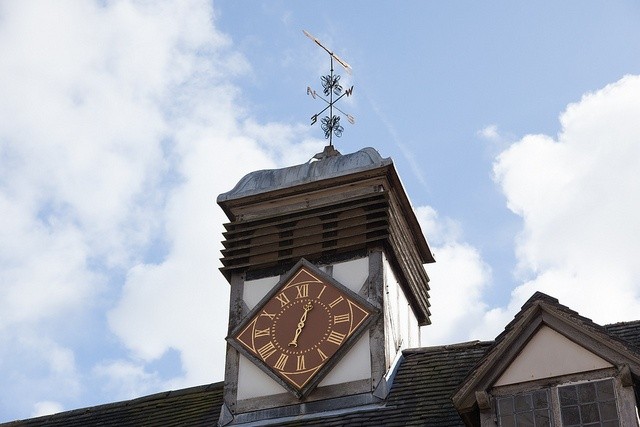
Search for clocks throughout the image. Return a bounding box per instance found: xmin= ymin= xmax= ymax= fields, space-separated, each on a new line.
xmin=225 ymin=258 xmax=383 ymax=401
